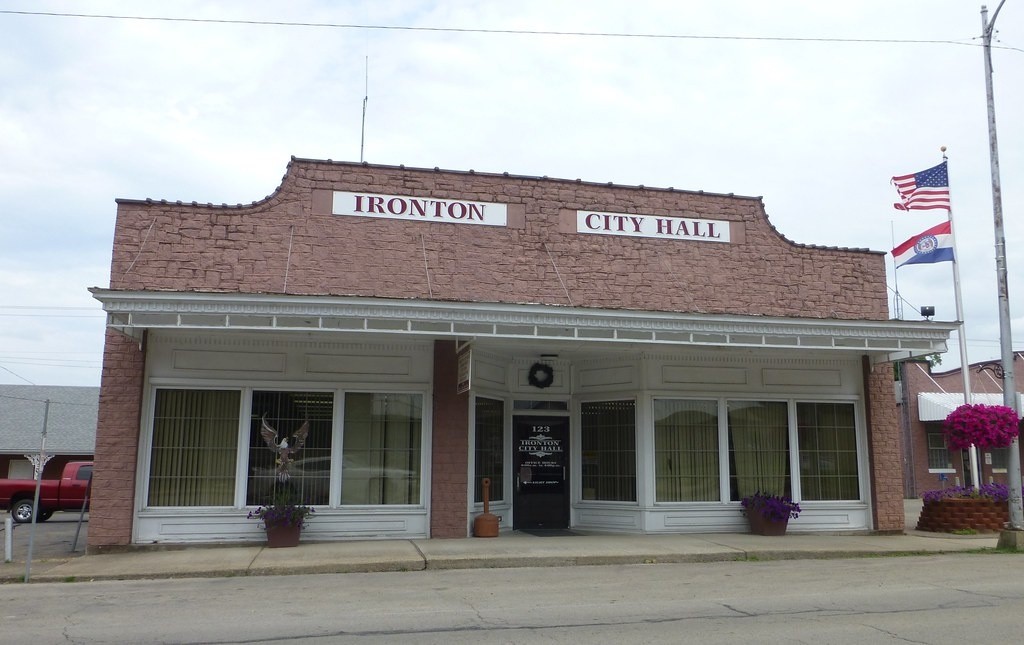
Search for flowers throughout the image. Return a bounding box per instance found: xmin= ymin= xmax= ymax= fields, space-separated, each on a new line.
xmin=738 ymin=489 xmax=802 ymax=520
xmin=247 ymin=490 xmax=316 ymax=531
xmin=942 ymin=403 xmax=1020 ymax=451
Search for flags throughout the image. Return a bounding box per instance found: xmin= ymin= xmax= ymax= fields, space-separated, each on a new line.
xmin=893 ymin=161 xmax=950 ymax=211
xmin=890 ymin=220 xmax=955 ymax=270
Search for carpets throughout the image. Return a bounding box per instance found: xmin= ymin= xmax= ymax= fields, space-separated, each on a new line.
xmin=519 ymin=529 xmax=588 ymax=538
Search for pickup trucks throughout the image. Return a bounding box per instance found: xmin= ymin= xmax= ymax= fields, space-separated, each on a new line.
xmin=0 ymin=461 xmax=94 ymax=524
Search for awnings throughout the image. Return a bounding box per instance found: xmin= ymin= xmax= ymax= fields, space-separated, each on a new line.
xmin=918 ymin=392 xmax=1024 ymax=420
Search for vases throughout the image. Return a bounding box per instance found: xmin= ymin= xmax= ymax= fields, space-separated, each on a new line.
xmin=264 ymin=522 xmax=301 ymax=548
xmin=749 ymin=510 xmax=789 ymax=536
